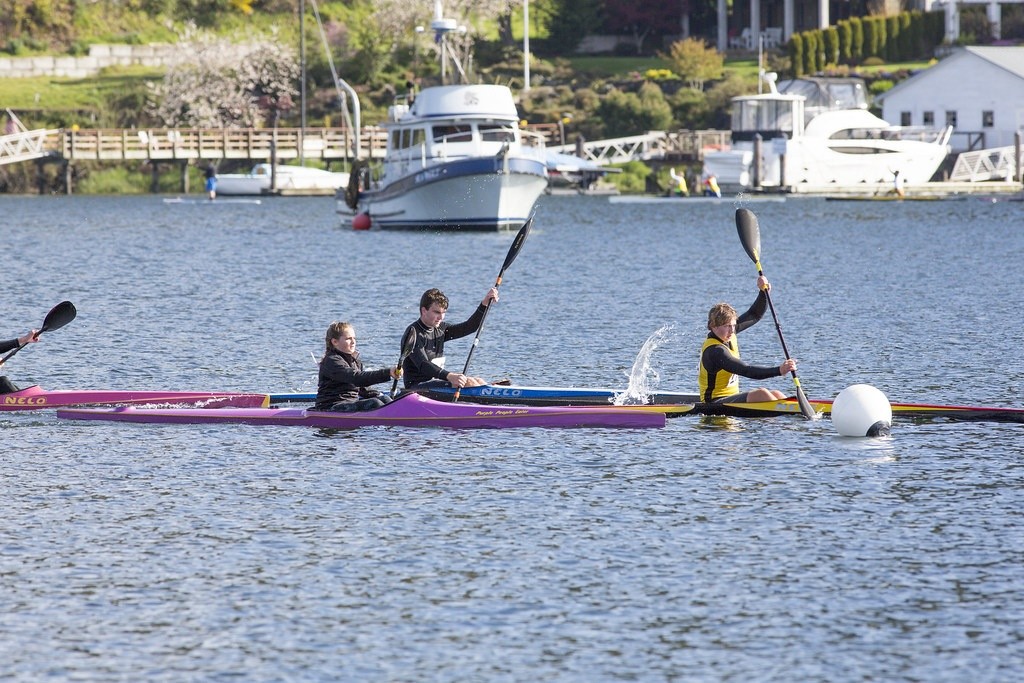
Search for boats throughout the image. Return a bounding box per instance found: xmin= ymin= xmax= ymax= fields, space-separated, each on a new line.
xmin=211 ymin=164 xmax=350 ymax=195
xmin=55 ymin=394 xmax=667 ymax=428
xmin=700 ymin=35 xmax=953 ymax=198
xmin=0 ymin=385 xmax=270 ymax=411
xmin=311 ymin=0 xmax=551 ymax=231
xmin=615 ymin=395 xmax=1024 ymax=422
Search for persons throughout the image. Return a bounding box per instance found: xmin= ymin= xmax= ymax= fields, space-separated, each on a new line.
xmin=316 ymin=321 xmax=404 ymax=411
xmin=705 ymin=174 xmax=720 ymax=196
xmin=198 ymin=162 xmax=218 ymax=200
xmin=699 ymin=276 xmax=797 ymax=403
xmin=0 ymin=330 xmax=39 ymax=393
xmin=407 ymin=82 xmax=415 ymax=109
xmin=670 ymin=168 xmax=688 ymax=196
xmin=400 ymin=288 xmax=498 ymax=388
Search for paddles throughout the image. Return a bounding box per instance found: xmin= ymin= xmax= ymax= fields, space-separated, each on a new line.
xmin=453 ymin=217 xmax=533 ymax=403
xmin=391 ymin=326 xmax=417 ymax=397
xmin=735 ymin=209 xmax=816 ymax=421
xmin=0 ymin=300 xmax=77 ymax=367
